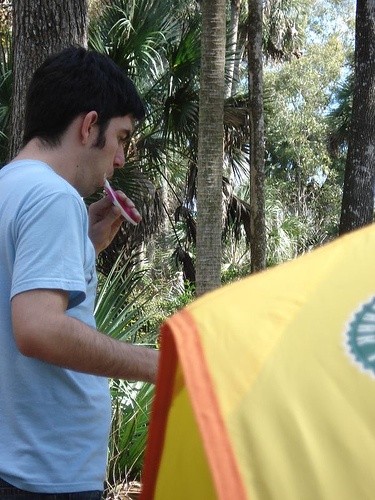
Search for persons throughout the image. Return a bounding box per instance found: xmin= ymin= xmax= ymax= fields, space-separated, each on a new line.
xmin=0 ymin=46 xmax=162 ymax=499
